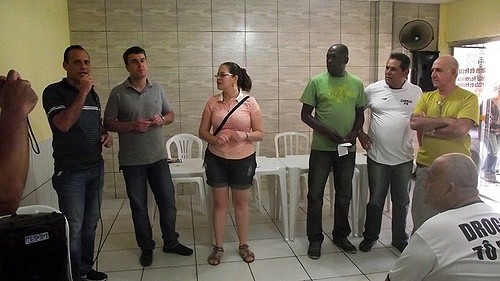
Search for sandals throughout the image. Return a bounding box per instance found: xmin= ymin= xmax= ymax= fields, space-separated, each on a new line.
xmin=208 ymin=246 xmax=224 ymax=265
xmin=239 ymin=245 xmax=255 ymax=263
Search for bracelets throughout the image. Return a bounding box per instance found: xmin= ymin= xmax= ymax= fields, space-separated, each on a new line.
xmin=245 ymin=131 xmax=249 ymax=140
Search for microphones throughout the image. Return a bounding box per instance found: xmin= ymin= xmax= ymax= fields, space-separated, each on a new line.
xmin=90 ymin=87 xmax=100 ymax=109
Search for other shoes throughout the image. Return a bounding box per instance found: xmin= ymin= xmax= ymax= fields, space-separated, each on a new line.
xmin=491 ymin=171 xmax=500 ymax=183
xmin=481 ymin=172 xmax=491 ymax=182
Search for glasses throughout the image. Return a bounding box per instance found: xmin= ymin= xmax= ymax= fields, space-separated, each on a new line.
xmin=215 ymin=72 xmax=234 ymax=78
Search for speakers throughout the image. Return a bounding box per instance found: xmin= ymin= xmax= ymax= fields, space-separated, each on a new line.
xmin=0 ymin=211 xmax=71 ymax=281
xmin=411 ymin=50 xmax=440 ymax=91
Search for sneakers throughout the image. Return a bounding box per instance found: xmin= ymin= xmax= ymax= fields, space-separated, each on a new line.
xmin=163 ymin=243 xmax=193 ymax=255
xmin=359 ymin=239 xmax=377 ymax=252
xmin=333 ymin=237 xmax=357 ymax=253
xmin=80 ymin=269 xmax=108 ymax=281
xmin=308 ymin=241 xmax=322 ymax=259
xmin=392 ymin=240 xmax=408 ymax=253
xmin=140 ymin=250 xmax=153 ymax=267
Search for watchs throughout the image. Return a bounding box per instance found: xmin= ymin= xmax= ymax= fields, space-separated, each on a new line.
xmin=160 ymin=116 xmax=165 ymax=123
xmin=430 ymin=129 xmax=436 ymax=137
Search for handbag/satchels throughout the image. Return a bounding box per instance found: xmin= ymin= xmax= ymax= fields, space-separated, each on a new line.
xmin=490 ymin=124 xmax=500 ymax=135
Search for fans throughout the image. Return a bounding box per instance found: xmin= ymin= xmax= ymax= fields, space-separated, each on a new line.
xmin=397 ymin=19 xmax=435 ymax=57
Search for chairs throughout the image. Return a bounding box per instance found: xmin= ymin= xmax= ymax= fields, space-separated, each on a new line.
xmin=0 ymin=206 xmax=73 ymax=281
xmin=165 ymin=133 xmax=207 ymax=218
xmin=228 ymin=140 xmax=262 ymax=215
xmin=273 ymin=131 xmax=311 ymax=222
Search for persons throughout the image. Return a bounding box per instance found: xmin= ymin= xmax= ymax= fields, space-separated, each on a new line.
xmin=199 ymin=62 xmax=265 ymax=265
xmin=410 ymin=55 xmax=479 ymax=239
xmin=481 ymin=84 xmax=500 ymax=184
xmin=299 ymin=43 xmax=366 ymax=258
xmin=43 ymin=45 xmax=113 ymax=281
xmin=385 ymin=152 xmax=500 ymax=281
xmin=103 ymin=46 xmax=194 ymax=266
xmin=357 ymin=53 xmax=425 ymax=252
xmin=0 ymin=68 xmax=38 ymax=216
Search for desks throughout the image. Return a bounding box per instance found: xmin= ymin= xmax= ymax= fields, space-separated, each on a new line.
xmin=243 ymin=154 xmax=289 ymax=242
xmin=147 ymin=157 xmax=212 ymax=229
xmin=354 ymin=151 xmax=368 ymax=239
xmin=279 ymin=153 xmax=359 ymax=242
